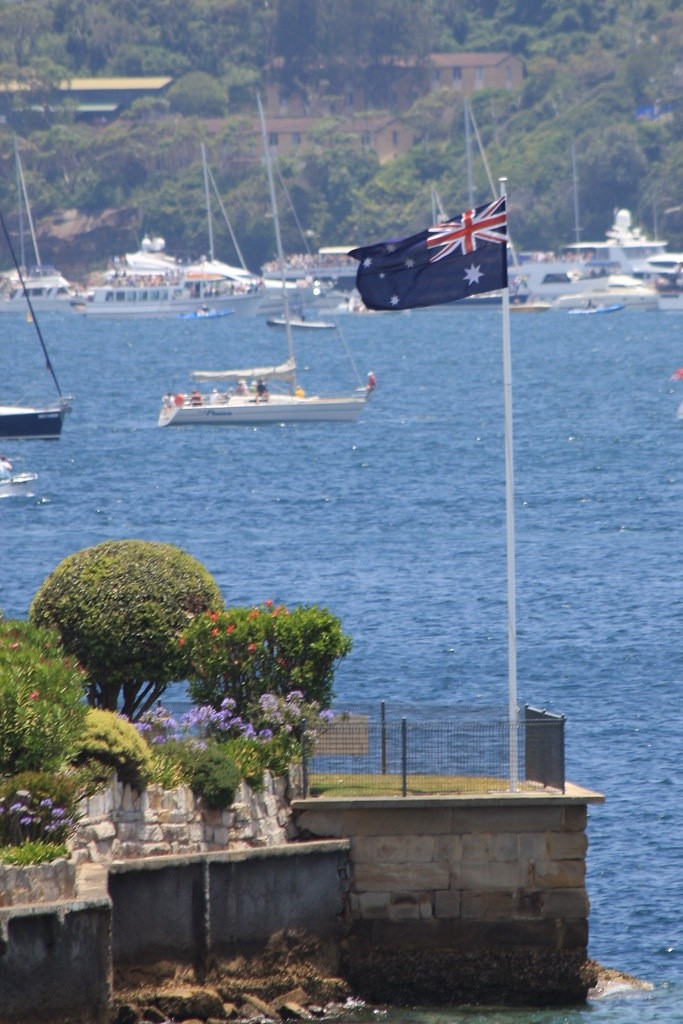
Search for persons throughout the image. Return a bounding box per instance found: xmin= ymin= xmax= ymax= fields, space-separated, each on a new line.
xmin=531 ymin=251 xmax=593 ymax=262
xmin=0 ymin=451 xmax=13 ymax=480
xmin=264 ymin=254 xmax=352 ymax=271
xmin=163 ymin=393 xmax=176 ymax=411
xmin=188 ymin=390 xmax=203 ymax=406
xmin=230 ymin=279 xmax=266 ymax=294
xmin=210 ymin=379 xmax=270 ymax=405
xmin=366 ymin=371 xmax=376 ymax=397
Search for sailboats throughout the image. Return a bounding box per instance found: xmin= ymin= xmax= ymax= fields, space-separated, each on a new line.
xmin=0 ymin=213 xmax=76 ymax=441
xmin=157 ymin=87 xmax=377 ymax=426
xmin=0 ymin=130 xmax=73 ymax=310
xmin=188 ymin=141 xmax=336 ymax=312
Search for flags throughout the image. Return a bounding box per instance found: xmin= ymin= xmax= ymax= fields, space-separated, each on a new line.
xmin=348 ymin=194 xmax=509 ymax=310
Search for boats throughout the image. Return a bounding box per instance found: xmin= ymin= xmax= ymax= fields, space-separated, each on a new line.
xmin=266 ymin=313 xmax=336 ymax=332
xmin=176 ymin=308 xmax=234 ymax=320
xmin=260 ymin=247 xmax=365 ymax=293
xmin=0 ymin=472 xmax=39 ymax=498
xmin=70 ymin=233 xmax=265 ymax=315
xmin=433 ymin=96 xmax=682 ymax=317
